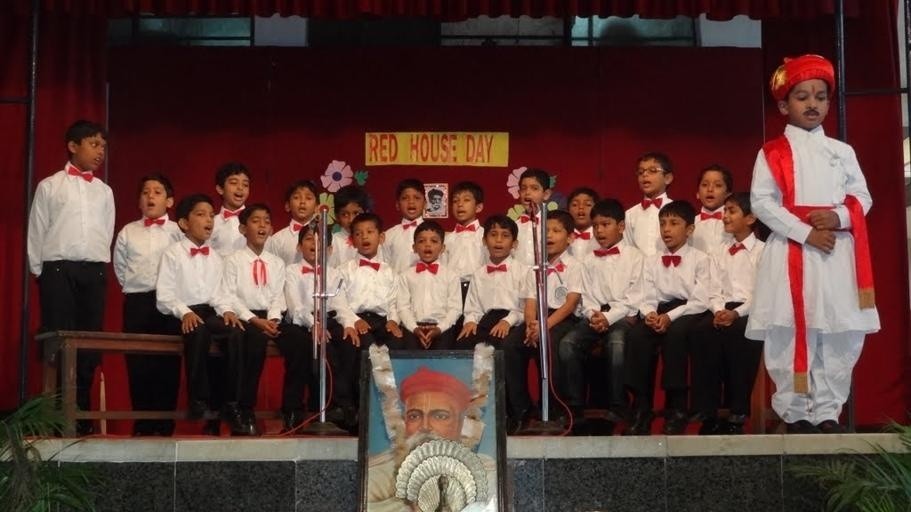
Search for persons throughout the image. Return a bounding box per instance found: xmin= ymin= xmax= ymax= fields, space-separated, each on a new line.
xmin=25 ymin=119 xmax=115 ymax=434
xmin=687 ymin=191 xmax=765 ymax=425
xmin=334 ymin=211 xmax=405 ymax=352
xmin=205 ymin=162 xmax=250 ymax=258
xmin=382 ymin=177 xmax=431 ymax=274
xmin=510 ymin=168 xmax=551 ymax=270
xmin=112 ymin=173 xmax=183 ymax=436
xmin=328 ymin=185 xmax=387 ymax=268
xmin=396 ymin=220 xmax=462 ymax=350
xmin=621 ymin=198 xmax=712 ymax=438
xmin=558 ymin=197 xmax=636 ymax=427
xmin=268 ymin=179 xmax=323 ymax=266
xmin=423 ymin=183 xmax=448 ymax=218
xmin=223 ymin=202 xmax=312 ymax=437
xmin=686 ymin=165 xmax=734 ymax=254
xmin=443 ymin=181 xmax=489 ymax=296
xmin=620 ymin=151 xmax=675 ymax=260
xmin=455 ymin=213 xmax=531 ymax=426
xmin=522 ymin=210 xmax=577 ymax=426
xmin=283 ymin=222 xmax=360 ymax=431
xmin=564 ymin=186 xmax=598 ymax=259
xmin=154 ymin=193 xmax=255 ymax=437
xmin=368 ymin=359 xmax=496 ymax=511
xmin=743 ymin=52 xmax=881 ymax=435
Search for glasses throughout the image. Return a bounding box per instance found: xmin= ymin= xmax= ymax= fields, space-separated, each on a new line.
xmin=637 ymin=166 xmax=667 ymax=176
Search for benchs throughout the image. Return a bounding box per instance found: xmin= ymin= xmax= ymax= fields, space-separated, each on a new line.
xmin=34 ymin=329 xmax=315 ymax=438
xmin=527 ymin=336 xmax=730 ymax=423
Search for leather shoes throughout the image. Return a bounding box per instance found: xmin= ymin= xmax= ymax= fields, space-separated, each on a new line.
xmin=820 ymin=420 xmax=847 ymax=431
xmin=228 ymin=405 xmax=259 ymax=436
xmin=663 ymin=412 xmax=689 ymax=435
xmin=701 ymin=417 xmax=722 ymax=434
xmin=326 ymin=406 xmax=350 ymax=434
xmin=727 ymin=416 xmax=746 ymax=433
xmin=788 ymin=418 xmax=814 ymax=436
xmin=283 ymin=408 xmax=302 ymax=430
xmin=54 ymin=414 xmax=98 ymax=441
xmin=201 ymin=411 xmax=221 ymax=434
xmin=624 ymin=408 xmax=654 ymax=434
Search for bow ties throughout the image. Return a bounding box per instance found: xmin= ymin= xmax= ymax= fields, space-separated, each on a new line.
xmin=144 ymin=218 xmax=164 ymax=226
xmin=548 ymin=264 xmax=563 ymax=273
xmin=456 ymin=225 xmax=476 ymax=232
xmin=191 ymin=247 xmax=212 ymax=254
xmin=293 ymin=222 xmax=305 ymax=230
xmin=662 ymin=255 xmax=681 ymax=268
xmin=361 ymin=258 xmax=381 ymax=271
xmin=641 ymin=200 xmax=663 ymax=211
xmin=594 ymin=247 xmax=618 ymax=255
xmin=403 ymin=222 xmax=416 ymax=229
xmin=575 ymin=231 xmax=590 ymax=238
xmin=416 ymin=262 xmax=438 ymax=273
xmin=487 ymin=265 xmax=506 ymax=272
xmin=701 ymin=211 xmax=721 ymax=221
xmin=729 ymin=244 xmax=745 ymax=254
xmin=68 ymin=165 xmax=95 ymax=182
xmin=224 ymin=211 xmax=240 ymax=218
xmin=520 ymin=216 xmax=538 ymax=224
xmin=303 ymin=266 xmax=320 ymax=273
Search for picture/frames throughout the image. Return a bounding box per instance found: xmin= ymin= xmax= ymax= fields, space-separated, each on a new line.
xmin=353 ymin=341 xmax=512 ymax=512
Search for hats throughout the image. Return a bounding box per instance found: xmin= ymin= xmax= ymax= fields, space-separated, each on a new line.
xmin=429 ymin=189 xmax=443 ymax=196
xmin=401 ymin=369 xmax=471 ymax=403
xmin=772 ymin=55 xmax=837 ymax=101
